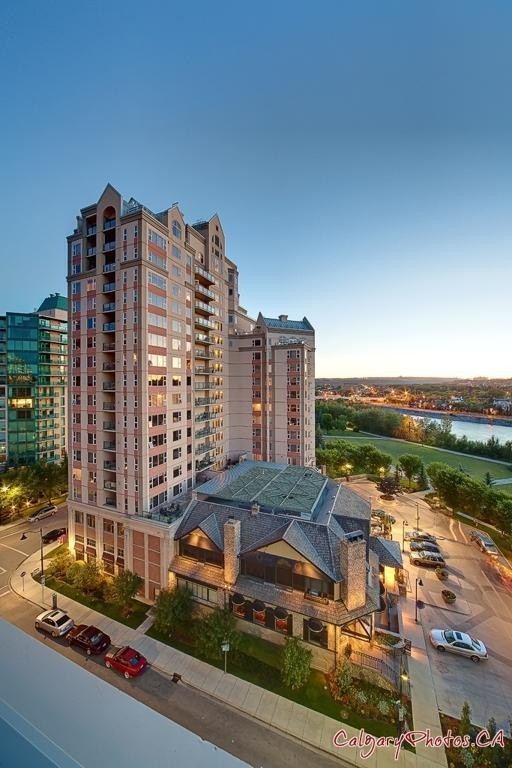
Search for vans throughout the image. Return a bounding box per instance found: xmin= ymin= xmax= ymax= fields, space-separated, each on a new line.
xmin=26 ymin=505 xmax=58 ymax=522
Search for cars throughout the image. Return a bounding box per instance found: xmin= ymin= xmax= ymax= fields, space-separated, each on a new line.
xmin=65 ymin=623 xmax=112 ymax=655
xmin=39 ymin=527 xmax=66 ymax=544
xmin=428 ymin=628 xmax=490 ymax=663
xmin=372 ymin=508 xmax=396 ymax=524
xmin=405 ymin=530 xmax=437 ymax=543
xmin=407 ymin=541 xmax=440 ymax=552
xmin=469 ymin=530 xmax=498 ymax=556
xmin=102 ymin=645 xmax=147 ymax=681
xmin=33 ymin=610 xmax=76 ymax=639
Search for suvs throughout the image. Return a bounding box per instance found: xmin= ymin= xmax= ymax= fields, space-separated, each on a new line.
xmin=409 ymin=550 xmax=448 ymax=568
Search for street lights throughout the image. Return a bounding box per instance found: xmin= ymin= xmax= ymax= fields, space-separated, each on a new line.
xmin=388 ymin=510 xmax=393 ymax=540
xmin=18 ymin=526 xmax=45 ymax=604
xmin=402 ymin=519 xmax=410 ymax=553
xmin=413 ymin=578 xmax=424 ymax=626
xmin=398 ymin=663 xmax=410 ymax=749
xmin=415 ymin=500 xmax=420 ymax=529
